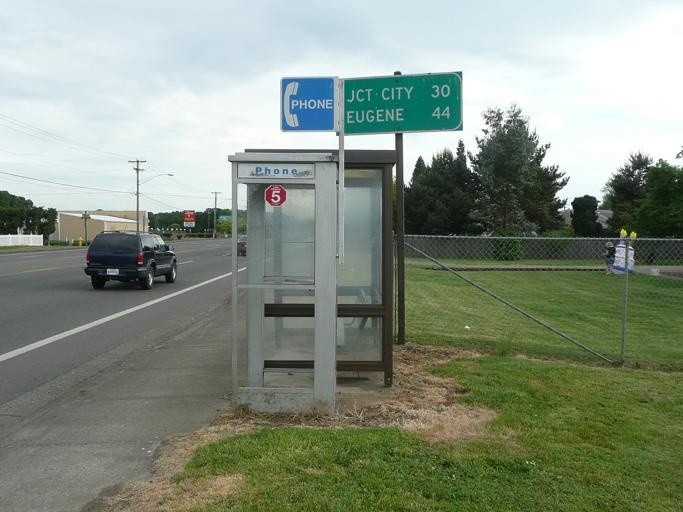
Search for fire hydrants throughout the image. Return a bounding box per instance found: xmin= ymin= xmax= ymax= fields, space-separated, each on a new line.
xmin=77 ymin=237 xmax=83 ymax=246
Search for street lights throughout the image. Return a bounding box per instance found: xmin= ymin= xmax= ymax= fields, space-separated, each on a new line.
xmin=136 ymin=172 xmax=174 ymax=232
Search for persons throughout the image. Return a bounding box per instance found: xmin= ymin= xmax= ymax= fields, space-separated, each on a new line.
xmin=602 ymin=241 xmax=616 ymax=276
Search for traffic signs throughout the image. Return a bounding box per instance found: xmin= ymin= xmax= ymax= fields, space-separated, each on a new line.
xmin=342 ymin=69 xmax=463 ymax=133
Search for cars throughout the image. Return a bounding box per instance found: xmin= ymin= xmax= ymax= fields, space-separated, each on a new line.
xmin=236 ymin=234 xmax=247 ymax=255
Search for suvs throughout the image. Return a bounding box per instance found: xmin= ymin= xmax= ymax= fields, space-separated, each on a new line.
xmin=84 ymin=229 xmax=180 ymax=290
